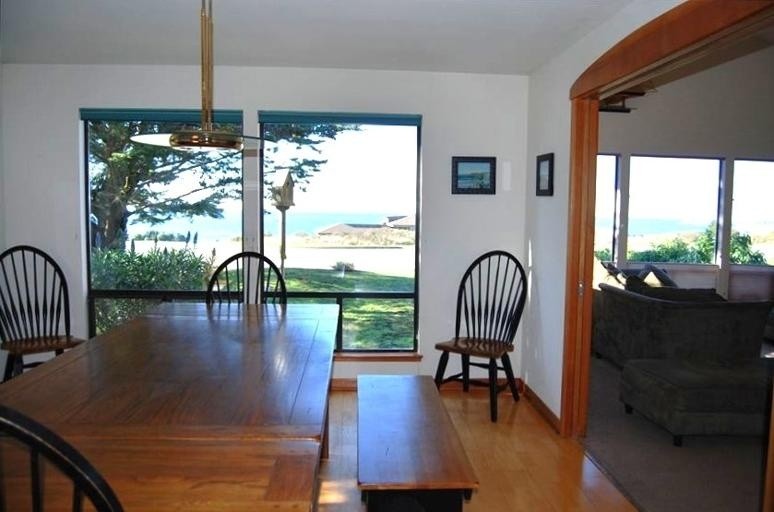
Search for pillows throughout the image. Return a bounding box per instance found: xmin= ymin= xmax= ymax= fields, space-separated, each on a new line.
xmin=607 ymin=263 xmax=726 ymax=302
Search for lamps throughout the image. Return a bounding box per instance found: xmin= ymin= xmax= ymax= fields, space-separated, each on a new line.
xmin=123 ymin=1 xmax=275 ymax=154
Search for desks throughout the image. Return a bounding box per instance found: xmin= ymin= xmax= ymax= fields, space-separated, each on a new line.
xmin=0 ymin=301 xmax=340 ymax=512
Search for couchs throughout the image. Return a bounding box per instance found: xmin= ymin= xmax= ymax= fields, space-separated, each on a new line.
xmin=591 ymin=261 xmax=773 ymax=367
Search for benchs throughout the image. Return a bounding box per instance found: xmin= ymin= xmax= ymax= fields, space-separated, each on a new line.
xmin=616 ymin=357 xmax=773 ymax=449
xmin=353 ymin=372 xmax=482 ymax=511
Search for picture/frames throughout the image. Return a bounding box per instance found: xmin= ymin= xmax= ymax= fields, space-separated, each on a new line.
xmin=534 ymin=152 xmax=554 ymax=198
xmin=450 ymin=155 xmax=495 ymax=196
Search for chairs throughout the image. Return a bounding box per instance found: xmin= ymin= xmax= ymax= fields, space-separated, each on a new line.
xmin=204 ymin=249 xmax=285 ymax=306
xmin=433 ymin=248 xmax=530 ymax=422
xmin=0 ymin=243 xmax=90 ymax=383
xmin=0 ymin=405 xmax=123 ymax=511
